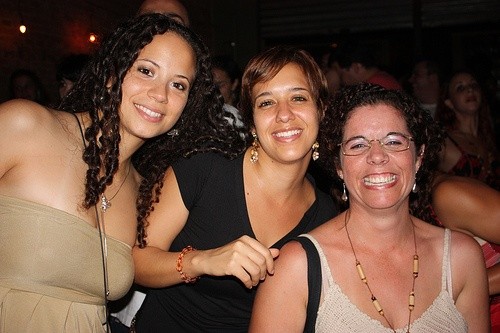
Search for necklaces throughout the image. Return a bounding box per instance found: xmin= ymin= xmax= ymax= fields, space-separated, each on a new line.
xmin=459 ymin=126 xmax=484 ymax=159
xmin=344 ymin=207 xmax=419 ymax=333
xmin=81 ymin=109 xmax=132 ymax=211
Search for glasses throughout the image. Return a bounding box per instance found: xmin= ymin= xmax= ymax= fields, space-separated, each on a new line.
xmin=337 ymin=136 xmax=418 ymax=156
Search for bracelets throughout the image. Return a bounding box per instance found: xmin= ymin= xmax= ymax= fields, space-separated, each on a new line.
xmin=176 ymin=245 xmax=202 ymax=283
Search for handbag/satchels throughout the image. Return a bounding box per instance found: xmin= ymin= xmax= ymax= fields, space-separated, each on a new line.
xmin=108 ymin=316 xmax=130 ymax=333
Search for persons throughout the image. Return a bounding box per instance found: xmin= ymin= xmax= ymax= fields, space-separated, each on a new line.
xmin=245 ymin=79 xmax=492 ymax=333
xmin=0 ymin=13 xmax=253 ymax=333
xmin=132 ymin=46 xmax=343 ymax=333
xmin=1 ymin=1 xmax=500 ymax=190
xmin=412 ymin=167 xmax=500 ymax=333
